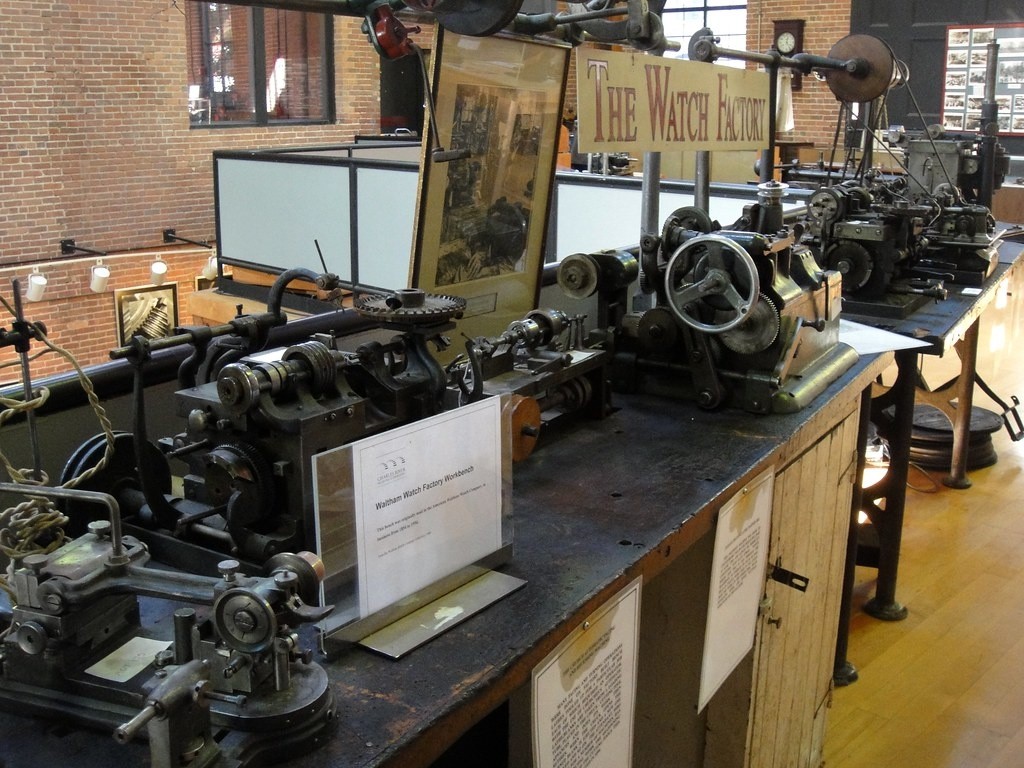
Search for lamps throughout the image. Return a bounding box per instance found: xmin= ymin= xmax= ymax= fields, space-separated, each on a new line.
xmin=25 ymin=267 xmax=48 ymax=302
xmin=201 ymin=249 xmax=224 ymax=280
xmin=90 ymin=258 xmax=111 ymax=295
xmin=151 ymin=254 xmax=168 ymax=285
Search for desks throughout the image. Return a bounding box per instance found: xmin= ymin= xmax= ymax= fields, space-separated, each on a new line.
xmin=841 ymin=238 xmax=1023 ymax=490
xmin=1 ymin=323 xmax=897 ymax=768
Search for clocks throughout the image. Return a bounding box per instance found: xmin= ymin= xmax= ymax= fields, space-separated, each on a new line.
xmin=771 ymin=19 xmax=806 ymax=92
